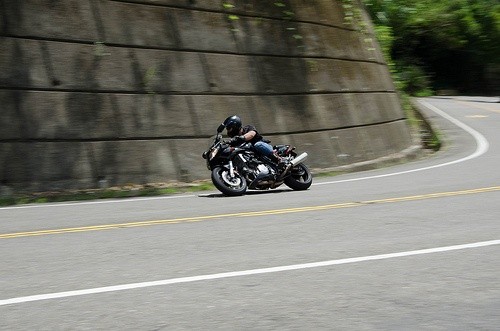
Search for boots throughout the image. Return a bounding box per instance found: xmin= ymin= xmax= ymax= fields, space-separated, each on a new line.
xmin=269 ymin=153 xmax=285 ymax=168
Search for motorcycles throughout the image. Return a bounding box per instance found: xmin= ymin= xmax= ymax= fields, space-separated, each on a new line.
xmin=202 ymin=123 xmax=312 ymax=196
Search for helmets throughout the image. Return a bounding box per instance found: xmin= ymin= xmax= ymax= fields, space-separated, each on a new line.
xmin=222 ymin=114 xmax=243 ymax=138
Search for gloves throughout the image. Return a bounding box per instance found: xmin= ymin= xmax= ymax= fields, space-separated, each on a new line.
xmin=224 ymin=136 xmax=244 ymax=148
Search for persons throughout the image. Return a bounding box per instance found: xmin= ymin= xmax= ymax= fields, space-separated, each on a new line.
xmin=224 ymin=116 xmax=291 ymax=176
